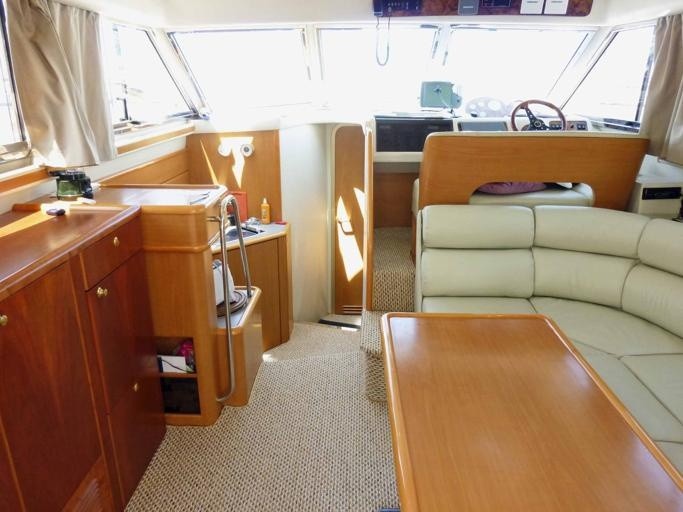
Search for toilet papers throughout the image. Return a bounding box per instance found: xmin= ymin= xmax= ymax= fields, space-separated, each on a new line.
xmin=241 ymin=142 xmax=255 ymax=157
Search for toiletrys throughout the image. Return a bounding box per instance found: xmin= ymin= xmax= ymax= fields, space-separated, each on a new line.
xmin=261 ymin=198 xmax=270 ymax=224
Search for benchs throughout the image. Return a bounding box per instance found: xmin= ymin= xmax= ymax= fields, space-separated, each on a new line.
xmin=411 ymin=131 xmax=651 ymax=263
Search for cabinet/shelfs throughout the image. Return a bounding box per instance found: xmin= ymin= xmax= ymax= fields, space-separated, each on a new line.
xmin=0 ymin=204 xmax=169 ymax=512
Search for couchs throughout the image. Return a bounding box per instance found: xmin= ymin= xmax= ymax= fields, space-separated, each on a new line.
xmin=414 ymin=204 xmax=683 ymax=475
